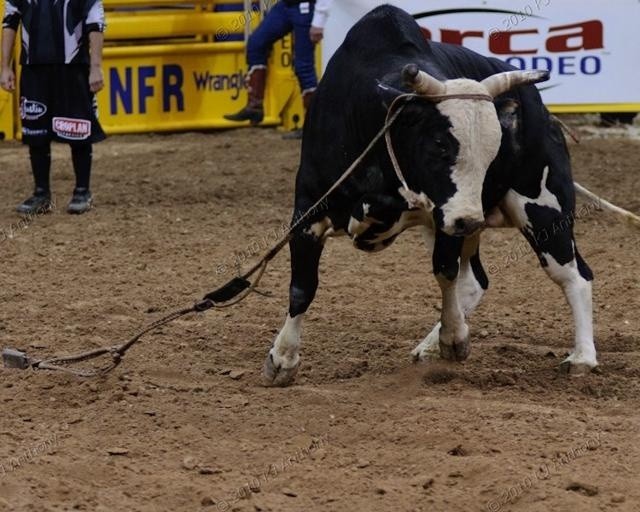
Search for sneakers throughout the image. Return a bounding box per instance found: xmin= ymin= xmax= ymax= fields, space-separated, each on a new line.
xmin=68 ymin=189 xmax=92 ymax=214
xmin=18 ymin=192 xmax=50 ymax=212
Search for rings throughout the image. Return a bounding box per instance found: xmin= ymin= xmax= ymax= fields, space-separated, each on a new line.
xmin=10 ymin=78 xmax=16 ymax=82
xmin=1 ymin=82 xmax=5 ymax=85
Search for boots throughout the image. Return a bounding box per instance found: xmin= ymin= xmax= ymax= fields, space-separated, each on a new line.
xmin=284 ymin=87 xmax=317 ymax=138
xmin=224 ymin=65 xmax=267 ymax=121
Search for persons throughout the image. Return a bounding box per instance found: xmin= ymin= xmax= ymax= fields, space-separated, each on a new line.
xmin=222 ymin=0 xmax=331 ymax=139
xmin=0 ymin=0 xmax=106 ymax=215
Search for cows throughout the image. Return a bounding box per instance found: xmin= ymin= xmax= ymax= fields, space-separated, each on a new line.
xmin=260 ymin=3 xmax=640 ymax=388
